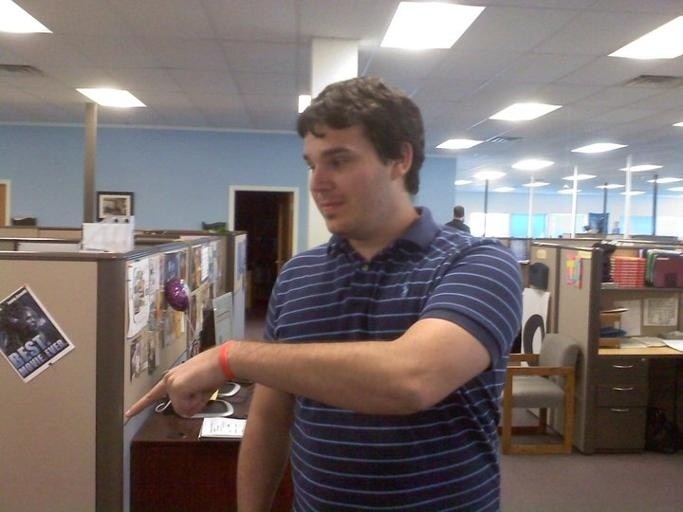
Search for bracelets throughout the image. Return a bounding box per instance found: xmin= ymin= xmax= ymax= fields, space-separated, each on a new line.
xmin=217 ymin=337 xmax=238 ymax=380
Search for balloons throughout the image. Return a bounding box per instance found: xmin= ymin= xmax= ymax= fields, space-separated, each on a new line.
xmin=164 ymin=276 xmax=193 ymax=312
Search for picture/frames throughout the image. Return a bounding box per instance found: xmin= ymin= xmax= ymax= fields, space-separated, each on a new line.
xmin=96 ymin=190 xmax=134 ymax=223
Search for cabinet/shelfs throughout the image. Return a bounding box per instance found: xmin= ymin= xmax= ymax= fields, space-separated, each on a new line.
xmin=592 ymin=281 xmax=683 ymax=452
xmin=130 ymin=375 xmax=295 ymax=512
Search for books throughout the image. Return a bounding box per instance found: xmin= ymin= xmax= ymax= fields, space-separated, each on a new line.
xmin=609 ymin=247 xmax=683 ymax=288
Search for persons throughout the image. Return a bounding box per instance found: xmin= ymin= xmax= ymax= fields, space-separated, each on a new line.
xmin=124 ymin=75 xmax=526 ymax=510
xmin=444 ymin=204 xmax=470 ymax=234
xmin=0 ymin=303 xmax=46 ymax=365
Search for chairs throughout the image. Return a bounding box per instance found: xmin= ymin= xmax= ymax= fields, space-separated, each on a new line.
xmin=496 ymin=333 xmax=580 ymax=454
